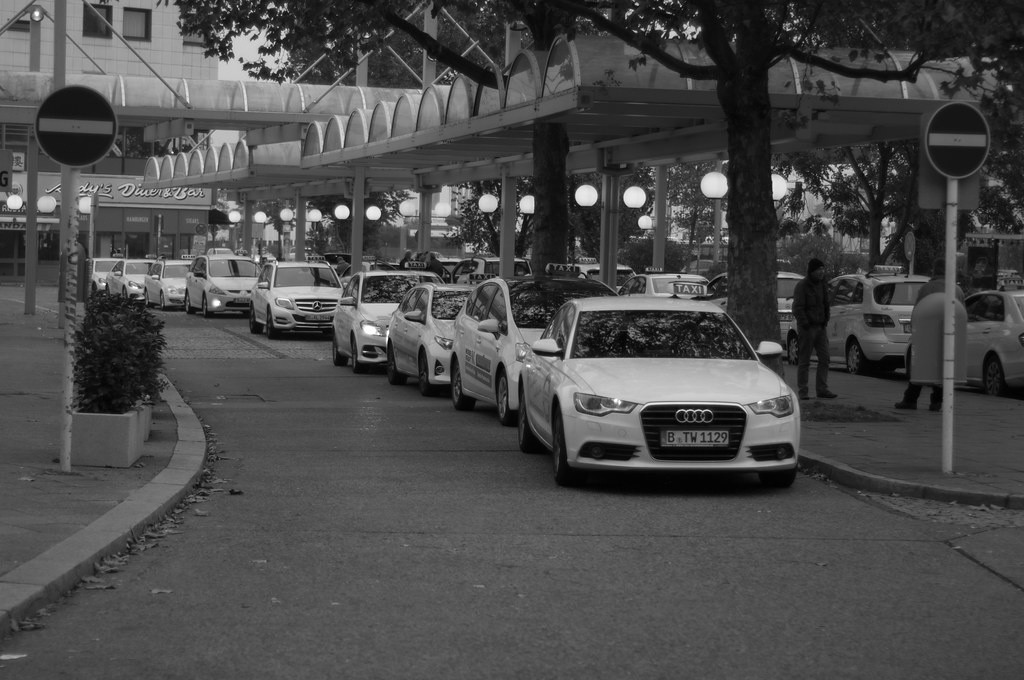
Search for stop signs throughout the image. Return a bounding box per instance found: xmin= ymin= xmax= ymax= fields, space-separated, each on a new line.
xmin=34 ymin=83 xmax=119 ymax=168
xmin=924 ymin=102 xmax=992 ymax=181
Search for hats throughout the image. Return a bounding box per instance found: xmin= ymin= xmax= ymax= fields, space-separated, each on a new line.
xmin=808 ymin=259 xmax=823 ymax=272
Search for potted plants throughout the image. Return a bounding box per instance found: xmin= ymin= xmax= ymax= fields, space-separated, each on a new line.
xmin=72 ymin=290 xmax=167 ymax=468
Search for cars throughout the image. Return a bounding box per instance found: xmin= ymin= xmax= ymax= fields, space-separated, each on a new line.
xmin=247 ymin=260 xmax=345 ymax=339
xmin=105 ymin=258 xmax=156 ymax=308
xmin=385 ymin=282 xmax=477 ymax=398
xmin=698 ymin=271 xmax=805 ymax=350
xmin=617 ymin=266 xmax=717 ymax=300
xmin=448 ymin=256 xmax=533 ymax=285
xmin=447 ymin=264 xmax=622 ymax=427
xmin=324 ymin=252 xmax=465 ymax=284
xmin=143 ymin=254 xmax=195 ymax=311
xmin=184 ymin=246 xmax=265 ymax=318
xmin=516 ymin=295 xmax=802 ymax=487
xmin=86 ymin=258 xmax=121 ymax=299
xmin=331 ymin=270 xmax=448 ymax=374
xmin=903 ymin=290 xmax=1024 ymax=399
xmin=565 ymin=255 xmax=635 ymax=294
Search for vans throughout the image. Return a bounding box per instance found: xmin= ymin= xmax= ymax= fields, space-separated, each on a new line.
xmin=785 ymin=264 xmax=937 ymax=375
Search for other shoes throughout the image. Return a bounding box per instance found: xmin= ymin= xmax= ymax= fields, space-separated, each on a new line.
xmin=800 ymin=391 xmax=809 ymax=400
xmin=816 ymin=389 xmax=838 ymax=399
xmin=894 ymin=399 xmax=917 ymax=410
xmin=929 ymin=402 xmax=941 ymax=412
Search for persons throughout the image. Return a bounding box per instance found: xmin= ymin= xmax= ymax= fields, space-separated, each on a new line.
xmin=894 ymin=253 xmax=966 ymax=411
xmin=336 ymin=257 xmax=351 ymax=277
xmin=792 ymin=258 xmax=838 ymax=400
xmin=399 ymin=250 xmax=443 ymax=278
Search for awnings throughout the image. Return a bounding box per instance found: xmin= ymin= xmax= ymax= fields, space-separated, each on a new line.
xmin=0 ymin=223 xmax=52 ymax=232
